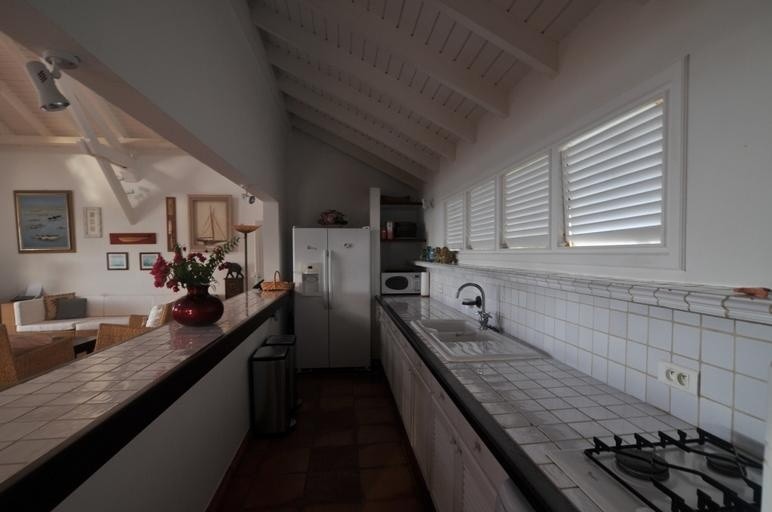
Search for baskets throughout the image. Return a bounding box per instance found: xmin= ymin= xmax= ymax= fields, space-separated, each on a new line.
xmin=260 ymin=269 xmax=293 ymax=291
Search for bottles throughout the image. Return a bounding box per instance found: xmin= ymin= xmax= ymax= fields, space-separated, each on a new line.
xmin=422 ymin=272 xmax=430 ymax=298
xmin=381 ymin=220 xmax=394 ymax=240
xmin=418 ymin=244 xmax=458 ymax=265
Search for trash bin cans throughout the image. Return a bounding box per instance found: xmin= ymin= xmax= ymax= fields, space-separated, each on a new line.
xmin=248 ymin=334 xmax=302 ymax=436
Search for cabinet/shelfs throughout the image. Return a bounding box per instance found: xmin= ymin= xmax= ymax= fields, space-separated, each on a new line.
xmin=375 ymin=299 xmax=533 ymax=512
xmin=379 ymin=200 xmax=428 ymax=244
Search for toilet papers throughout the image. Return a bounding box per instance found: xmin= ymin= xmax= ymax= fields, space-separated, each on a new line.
xmin=420 ymin=271 xmax=430 ymax=296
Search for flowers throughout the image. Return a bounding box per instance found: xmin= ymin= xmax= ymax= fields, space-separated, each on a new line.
xmin=316 ymin=208 xmax=348 ymax=223
xmin=150 ymin=236 xmax=247 ymax=290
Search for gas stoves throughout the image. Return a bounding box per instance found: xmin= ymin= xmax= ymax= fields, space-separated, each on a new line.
xmin=547 ymin=423 xmax=762 ymax=511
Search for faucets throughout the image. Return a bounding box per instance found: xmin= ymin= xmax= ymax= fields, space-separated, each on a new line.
xmin=456 ymin=282 xmax=488 ymax=330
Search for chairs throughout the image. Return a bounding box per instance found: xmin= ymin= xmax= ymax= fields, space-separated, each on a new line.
xmin=1 ymin=284 xmax=177 ymax=390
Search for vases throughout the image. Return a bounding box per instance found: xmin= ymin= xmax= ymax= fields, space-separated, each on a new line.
xmin=173 ymin=283 xmax=224 ymax=325
xmin=321 ymin=223 xmax=343 ymax=230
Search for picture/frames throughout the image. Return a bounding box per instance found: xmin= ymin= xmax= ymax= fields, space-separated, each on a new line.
xmin=13 ymin=188 xmax=78 ymax=256
xmin=188 ymin=191 xmax=235 ymax=252
xmin=105 ymin=252 xmax=130 ymax=270
xmin=83 ymin=206 xmax=104 ymax=238
xmin=140 ymin=251 xmax=160 ymax=271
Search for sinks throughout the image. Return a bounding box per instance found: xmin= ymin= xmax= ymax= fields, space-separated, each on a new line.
xmin=433 ymin=332 xmax=497 ymax=343
xmin=422 ymin=321 xmax=477 ymax=332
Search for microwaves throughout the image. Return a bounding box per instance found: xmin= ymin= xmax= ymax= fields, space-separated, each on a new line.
xmin=381 ymin=272 xmax=421 ymax=296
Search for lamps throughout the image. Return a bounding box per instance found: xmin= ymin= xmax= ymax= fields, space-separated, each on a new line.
xmin=238 ymin=184 xmax=256 ymax=204
xmin=24 ymin=49 xmax=81 ymax=113
xmin=234 ymin=224 xmax=262 ymax=292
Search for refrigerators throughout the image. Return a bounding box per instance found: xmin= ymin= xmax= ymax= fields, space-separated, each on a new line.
xmin=291 ymin=226 xmax=372 ymax=368
